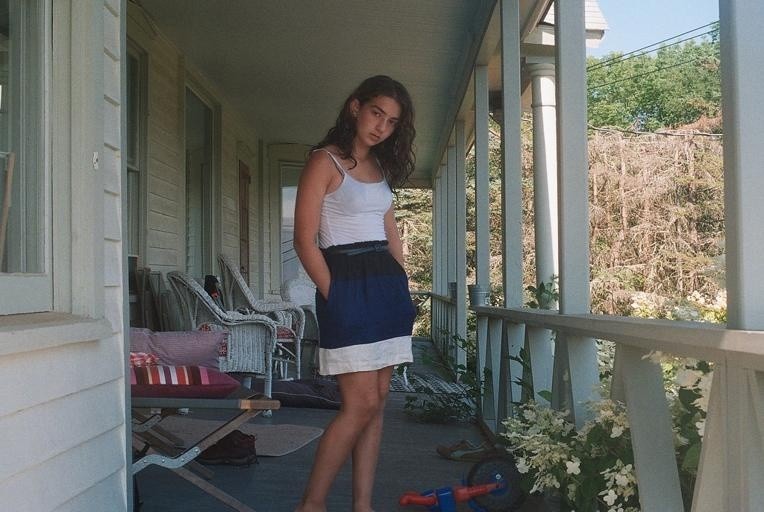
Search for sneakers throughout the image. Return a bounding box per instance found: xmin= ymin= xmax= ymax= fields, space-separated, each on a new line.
xmin=194 ymin=430 xmax=258 ymax=468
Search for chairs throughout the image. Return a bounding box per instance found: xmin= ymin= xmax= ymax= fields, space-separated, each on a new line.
xmin=161 ymin=264 xmax=278 ymax=417
xmin=278 ymin=278 xmax=322 ymax=369
xmin=215 ymin=251 xmax=305 ymax=383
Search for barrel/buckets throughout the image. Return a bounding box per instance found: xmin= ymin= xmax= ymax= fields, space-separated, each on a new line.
xmin=446 ymin=281 xmax=457 ymax=300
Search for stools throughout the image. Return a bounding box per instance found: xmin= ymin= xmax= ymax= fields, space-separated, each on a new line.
xmin=124 ymin=383 xmax=283 ymax=509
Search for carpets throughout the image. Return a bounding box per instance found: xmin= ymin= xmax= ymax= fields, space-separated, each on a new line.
xmin=320 ymin=371 xmax=467 ymax=394
xmin=131 ymin=412 xmax=325 ymax=456
xmin=248 ymin=376 xmax=346 ymax=410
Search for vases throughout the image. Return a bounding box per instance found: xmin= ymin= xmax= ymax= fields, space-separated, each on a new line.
xmin=447 ymin=281 xmax=456 ymax=298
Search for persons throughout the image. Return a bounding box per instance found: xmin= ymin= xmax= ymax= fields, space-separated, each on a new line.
xmin=292 ymin=74 xmax=416 ymax=512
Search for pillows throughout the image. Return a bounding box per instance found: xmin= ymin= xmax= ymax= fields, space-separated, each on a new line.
xmin=128 ymin=324 xmax=227 ymax=373
xmin=129 ymin=354 xmax=240 ymax=399
